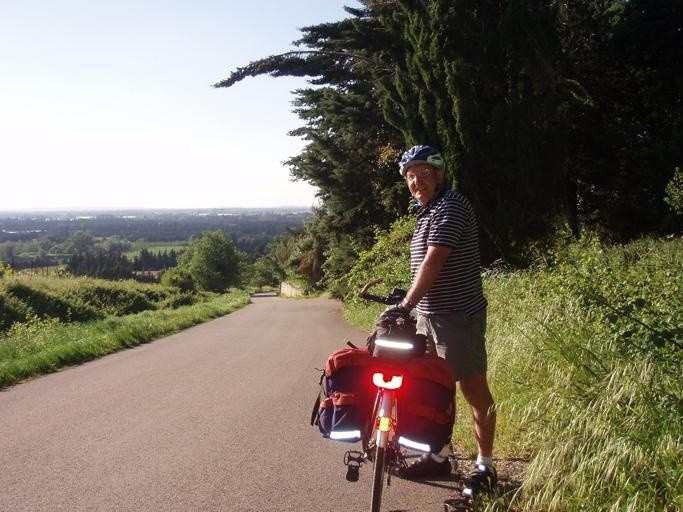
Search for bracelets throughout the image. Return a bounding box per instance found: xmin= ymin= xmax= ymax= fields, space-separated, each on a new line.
xmin=402 ymin=297 xmax=416 ymax=310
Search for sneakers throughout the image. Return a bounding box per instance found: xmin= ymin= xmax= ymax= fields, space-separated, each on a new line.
xmin=463 ymin=463 xmax=498 ymax=499
xmin=400 ymin=451 xmax=453 ymax=478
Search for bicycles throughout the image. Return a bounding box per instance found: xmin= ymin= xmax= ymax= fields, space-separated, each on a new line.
xmin=343 ymin=269 xmax=429 ymax=512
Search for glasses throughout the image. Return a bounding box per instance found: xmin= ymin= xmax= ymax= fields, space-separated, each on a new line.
xmin=403 ymin=168 xmax=436 ymax=179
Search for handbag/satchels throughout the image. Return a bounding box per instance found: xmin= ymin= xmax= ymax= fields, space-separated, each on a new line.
xmin=311 ymin=347 xmax=372 ymax=444
xmin=392 ymin=355 xmax=457 ymax=457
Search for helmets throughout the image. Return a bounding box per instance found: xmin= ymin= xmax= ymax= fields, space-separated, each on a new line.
xmin=398 ymin=143 xmax=446 ymax=178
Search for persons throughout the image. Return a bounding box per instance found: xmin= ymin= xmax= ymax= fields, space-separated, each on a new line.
xmin=376 ymin=143 xmax=498 ymax=498
